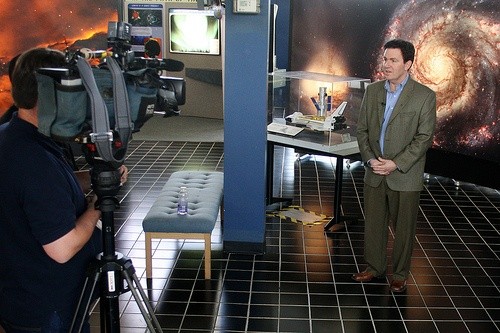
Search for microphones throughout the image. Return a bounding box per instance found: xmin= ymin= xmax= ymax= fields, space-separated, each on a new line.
xmin=159 ymin=58 xmax=184 ymax=72
xmin=381 ymin=102 xmax=386 ymax=106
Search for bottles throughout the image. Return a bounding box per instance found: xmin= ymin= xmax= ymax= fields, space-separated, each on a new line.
xmin=177 ymin=187 xmax=188 ymax=215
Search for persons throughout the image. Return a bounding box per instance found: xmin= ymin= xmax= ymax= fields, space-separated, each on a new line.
xmin=0 ymin=47 xmax=127 ymax=333
xmin=351 ymin=40 xmax=436 ymax=293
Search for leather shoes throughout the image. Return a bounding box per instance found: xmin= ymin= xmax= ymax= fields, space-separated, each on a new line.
xmin=391 ymin=280 xmax=407 ymax=292
xmin=352 ymin=271 xmax=377 ymax=282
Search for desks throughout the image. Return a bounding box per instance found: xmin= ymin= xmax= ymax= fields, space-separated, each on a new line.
xmin=267 ymin=132 xmax=361 ymax=231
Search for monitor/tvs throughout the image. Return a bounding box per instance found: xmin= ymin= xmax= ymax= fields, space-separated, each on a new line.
xmin=165 ymin=2 xmax=222 ymax=70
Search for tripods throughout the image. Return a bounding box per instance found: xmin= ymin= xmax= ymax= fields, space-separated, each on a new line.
xmin=69 ymin=162 xmax=163 ymax=333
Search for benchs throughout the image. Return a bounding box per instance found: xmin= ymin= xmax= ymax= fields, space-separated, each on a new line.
xmin=143 ymin=170 xmax=224 ymax=280
